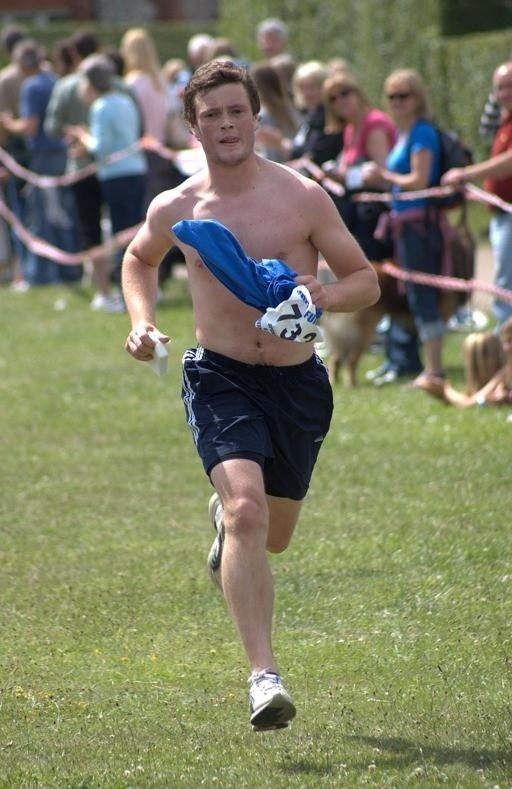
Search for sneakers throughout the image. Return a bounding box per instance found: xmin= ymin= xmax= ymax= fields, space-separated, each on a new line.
xmin=207 ymin=491 xmax=227 ymax=593
xmin=371 ymin=368 xmax=417 ymax=386
xmin=90 ymin=291 xmax=126 ymax=312
xmin=246 ymin=666 xmax=297 ymax=731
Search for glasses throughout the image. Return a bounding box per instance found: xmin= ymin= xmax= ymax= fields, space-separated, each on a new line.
xmin=384 ymin=91 xmax=413 ymax=101
xmin=329 ymin=90 xmax=349 ymax=102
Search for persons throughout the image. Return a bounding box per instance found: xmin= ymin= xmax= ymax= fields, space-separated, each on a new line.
xmin=120 ymin=60 xmax=381 ymax=728
xmin=1 ymin=19 xmax=299 ymax=313
xmin=255 ymin=51 xmax=512 ymax=410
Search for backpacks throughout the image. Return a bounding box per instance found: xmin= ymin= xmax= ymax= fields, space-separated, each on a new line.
xmin=406 ymin=119 xmax=473 ymax=207
